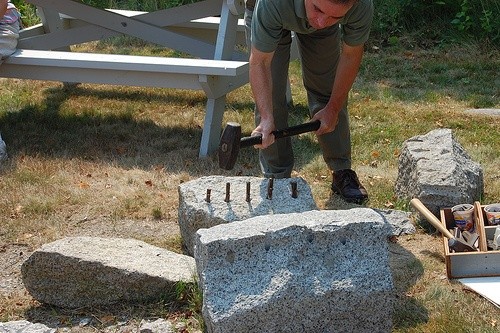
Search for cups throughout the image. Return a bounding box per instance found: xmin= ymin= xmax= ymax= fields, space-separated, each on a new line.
xmin=483 ymin=203 xmax=500 ymax=225
xmin=451 ymin=204 xmax=475 ymax=231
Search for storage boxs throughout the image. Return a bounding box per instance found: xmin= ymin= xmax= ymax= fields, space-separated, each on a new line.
xmin=438 ymin=204 xmax=500 ymax=279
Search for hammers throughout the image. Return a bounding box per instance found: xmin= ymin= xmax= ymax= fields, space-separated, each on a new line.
xmin=410 ymin=197 xmax=477 ymax=252
xmin=216 ymin=120 xmax=321 ymax=170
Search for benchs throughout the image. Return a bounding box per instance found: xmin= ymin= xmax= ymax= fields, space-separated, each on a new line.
xmin=0 ymin=7 xmax=300 ymax=102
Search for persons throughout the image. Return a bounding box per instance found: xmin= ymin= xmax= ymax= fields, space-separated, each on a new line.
xmin=244 ymin=0 xmax=375 ymax=203
xmin=0 ymin=0 xmax=21 ymax=162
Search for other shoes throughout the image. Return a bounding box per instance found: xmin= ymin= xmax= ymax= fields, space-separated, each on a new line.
xmin=0 ymin=136 xmax=8 ymax=160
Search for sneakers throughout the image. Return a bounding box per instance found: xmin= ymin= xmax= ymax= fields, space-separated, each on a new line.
xmin=332 ymin=169 xmax=368 ymax=204
